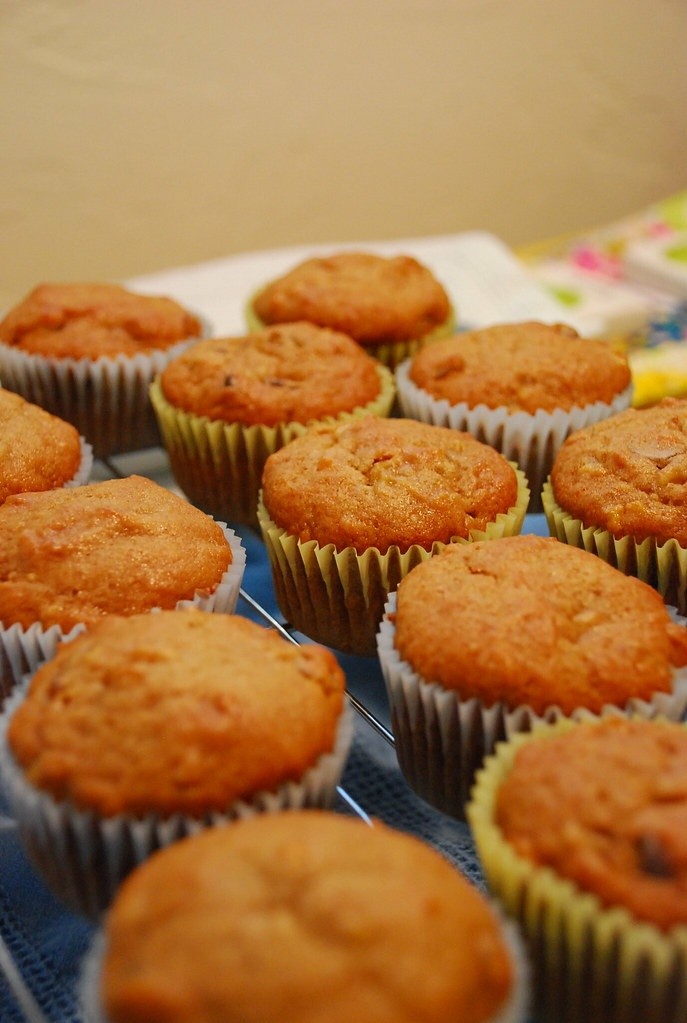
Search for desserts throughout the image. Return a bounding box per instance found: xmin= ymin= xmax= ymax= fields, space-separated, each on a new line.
xmin=0 ymin=250 xmax=686 ymax=1023
